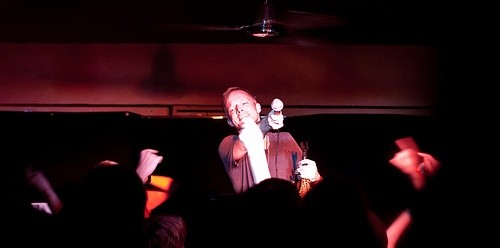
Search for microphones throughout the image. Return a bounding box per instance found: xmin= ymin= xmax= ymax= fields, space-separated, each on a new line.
xmin=270 ymin=98 xmax=284 ymax=115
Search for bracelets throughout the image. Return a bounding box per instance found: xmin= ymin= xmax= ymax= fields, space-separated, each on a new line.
xmin=259 ymin=116 xmax=272 ymax=138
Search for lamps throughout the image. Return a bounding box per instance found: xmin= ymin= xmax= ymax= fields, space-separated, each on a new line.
xmin=251 ymin=0 xmax=278 ymax=37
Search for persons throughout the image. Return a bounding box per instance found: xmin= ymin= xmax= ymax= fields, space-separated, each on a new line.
xmin=0 ymin=142 xmax=500 ymax=248
xmin=218 ymin=87 xmax=322 ymax=194
